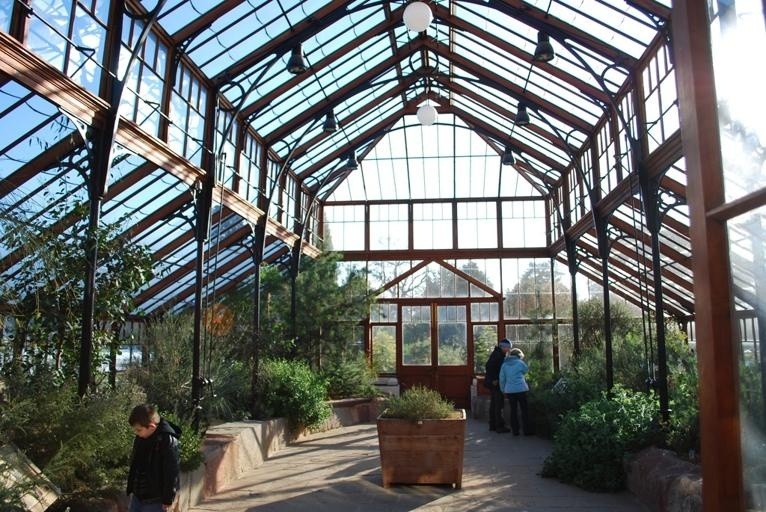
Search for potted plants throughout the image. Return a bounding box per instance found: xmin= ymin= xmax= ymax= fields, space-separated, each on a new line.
xmin=374 ymin=381 xmax=468 ymax=492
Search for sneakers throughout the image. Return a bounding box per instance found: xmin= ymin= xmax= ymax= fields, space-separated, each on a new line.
xmin=513 ymin=432 xmax=519 ymax=436
xmin=496 ymin=427 xmax=511 ymax=433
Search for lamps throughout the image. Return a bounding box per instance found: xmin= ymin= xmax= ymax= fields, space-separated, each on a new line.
xmin=500 ymin=147 xmax=516 ymax=165
xmin=345 ymin=149 xmax=358 ymax=170
xmin=415 ymin=74 xmax=441 ymax=126
xmin=513 ymin=100 xmax=530 ymax=126
xmin=321 ymin=107 xmax=339 ymax=132
xmin=402 ymin=0 xmax=434 ymax=33
xmin=531 ymin=26 xmax=555 ymax=62
xmin=284 ymin=39 xmax=309 ymax=75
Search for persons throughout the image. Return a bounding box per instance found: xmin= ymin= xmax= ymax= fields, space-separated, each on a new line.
xmin=124 ymin=403 xmax=182 ymax=512
xmin=498 ymin=347 xmax=536 ymax=435
xmin=483 ymin=338 xmax=512 ymax=432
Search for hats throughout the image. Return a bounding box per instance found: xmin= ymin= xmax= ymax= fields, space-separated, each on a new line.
xmin=499 ymin=339 xmax=512 ymax=348
